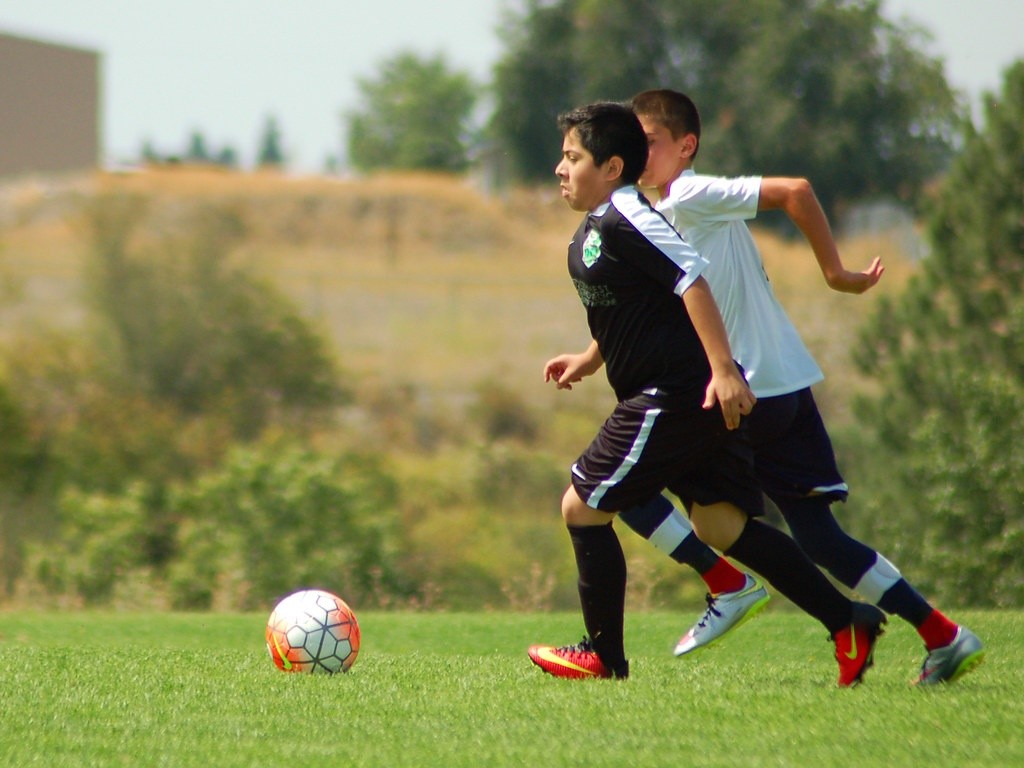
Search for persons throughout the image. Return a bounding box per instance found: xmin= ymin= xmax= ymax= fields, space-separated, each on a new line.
xmin=616 ymin=85 xmax=987 ymax=685
xmin=524 ymin=100 xmax=890 ymax=688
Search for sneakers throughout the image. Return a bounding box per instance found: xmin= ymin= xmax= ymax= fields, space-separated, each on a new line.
xmin=913 ymin=625 xmax=985 ymax=685
xmin=673 ymin=572 xmax=771 ymax=657
xmin=827 ymin=602 xmax=888 ymax=686
xmin=528 ymin=636 xmax=629 ymax=680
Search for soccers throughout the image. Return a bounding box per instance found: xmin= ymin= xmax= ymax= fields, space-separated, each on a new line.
xmin=264 ymin=588 xmax=362 ymax=677
